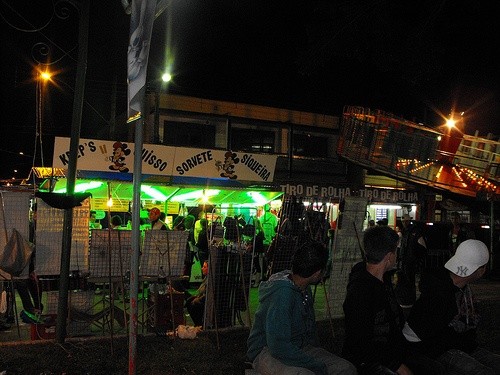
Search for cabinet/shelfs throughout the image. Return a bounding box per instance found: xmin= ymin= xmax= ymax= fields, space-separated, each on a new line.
xmin=445 ymin=209 xmax=473 ymax=223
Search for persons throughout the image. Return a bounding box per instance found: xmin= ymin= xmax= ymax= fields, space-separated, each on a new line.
xmin=172 ymin=260 xmax=209 ymax=327
xmin=367 ymin=215 xmax=467 ymax=273
xmin=246 ymin=241 xmax=358 ymax=375
xmin=343 ymin=225 xmax=440 ymax=375
xmin=411 ymin=239 xmax=500 ymax=375
xmin=89 ymin=207 xmax=268 ymax=287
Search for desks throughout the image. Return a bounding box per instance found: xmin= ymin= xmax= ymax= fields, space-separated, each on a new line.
xmin=0 ymin=271 xmax=89 ymax=337
xmin=86 ymin=274 xmax=190 ymax=359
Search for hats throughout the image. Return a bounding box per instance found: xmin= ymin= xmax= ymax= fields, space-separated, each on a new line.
xmin=401 ymin=214 xmax=413 ymax=221
xmin=444 ymin=239 xmax=488 ymax=277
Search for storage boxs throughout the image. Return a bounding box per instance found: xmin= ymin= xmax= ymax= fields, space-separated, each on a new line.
xmin=147 ymin=287 xmax=186 ymax=335
xmin=31 ymin=322 xmax=58 ymax=340
